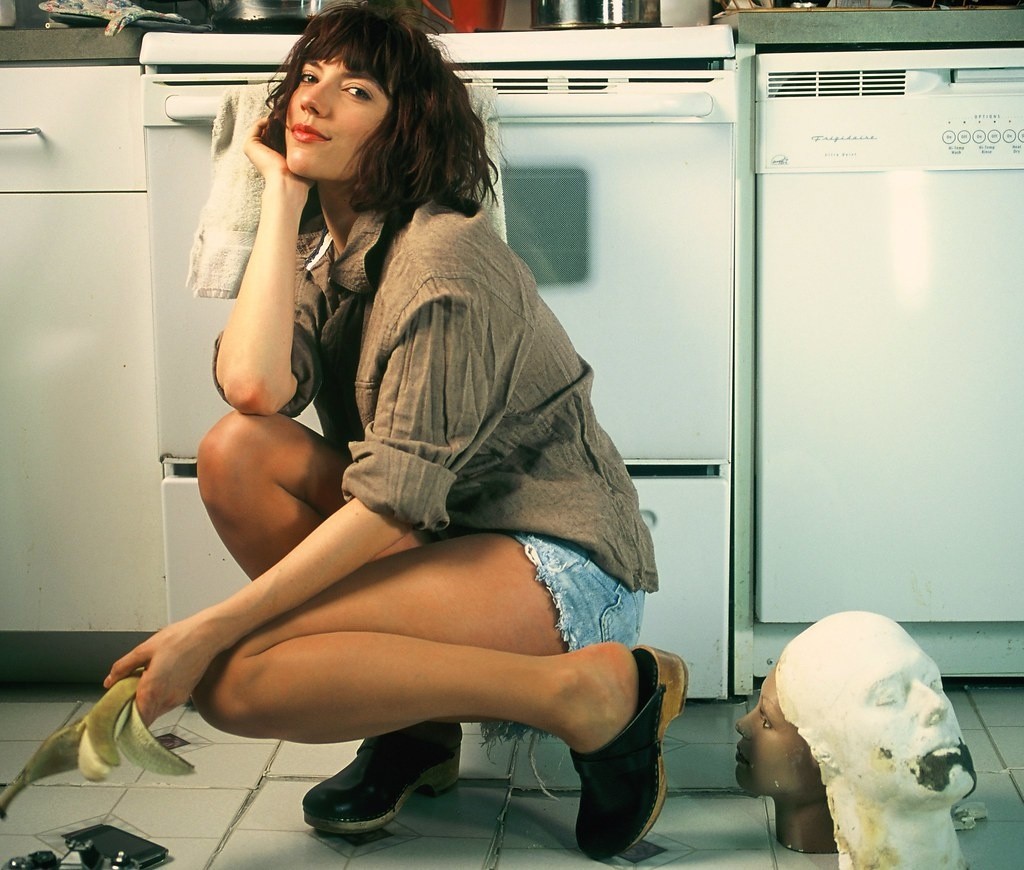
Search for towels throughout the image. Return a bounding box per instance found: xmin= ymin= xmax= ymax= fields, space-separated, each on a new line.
xmin=189 ymin=85 xmax=509 ymax=301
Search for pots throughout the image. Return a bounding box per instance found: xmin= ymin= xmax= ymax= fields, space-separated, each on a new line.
xmin=529 ymin=0 xmax=661 ymax=30
xmin=197 ymin=0 xmax=322 ymax=34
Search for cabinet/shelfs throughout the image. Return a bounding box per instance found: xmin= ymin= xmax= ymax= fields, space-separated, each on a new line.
xmin=0 ymin=28 xmax=169 ymax=687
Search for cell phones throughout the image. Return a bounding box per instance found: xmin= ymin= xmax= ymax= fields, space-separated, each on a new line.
xmin=65 ymin=824 xmax=169 ymax=870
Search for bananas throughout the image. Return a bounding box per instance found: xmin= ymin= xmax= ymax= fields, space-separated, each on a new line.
xmin=0 ymin=666 xmax=197 ymax=819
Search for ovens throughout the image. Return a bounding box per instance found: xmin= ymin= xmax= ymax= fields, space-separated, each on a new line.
xmin=123 ymin=32 xmax=747 ymax=703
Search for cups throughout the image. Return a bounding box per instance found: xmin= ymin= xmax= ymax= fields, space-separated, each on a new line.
xmin=660 ymin=0 xmax=710 ymax=27
xmin=1 ymin=2 xmax=17 ymax=27
xmin=419 ymin=0 xmax=507 ymax=33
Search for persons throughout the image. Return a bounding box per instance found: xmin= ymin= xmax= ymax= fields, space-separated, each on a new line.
xmin=735 ymin=665 xmax=838 ymax=853
xmin=776 ymin=611 xmax=976 ymax=869
xmin=104 ymin=3 xmax=689 ymax=860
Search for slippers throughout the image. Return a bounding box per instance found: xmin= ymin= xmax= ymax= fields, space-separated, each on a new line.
xmin=297 ymin=724 xmax=464 ymax=838
xmin=565 ymin=640 xmax=693 ymax=864
